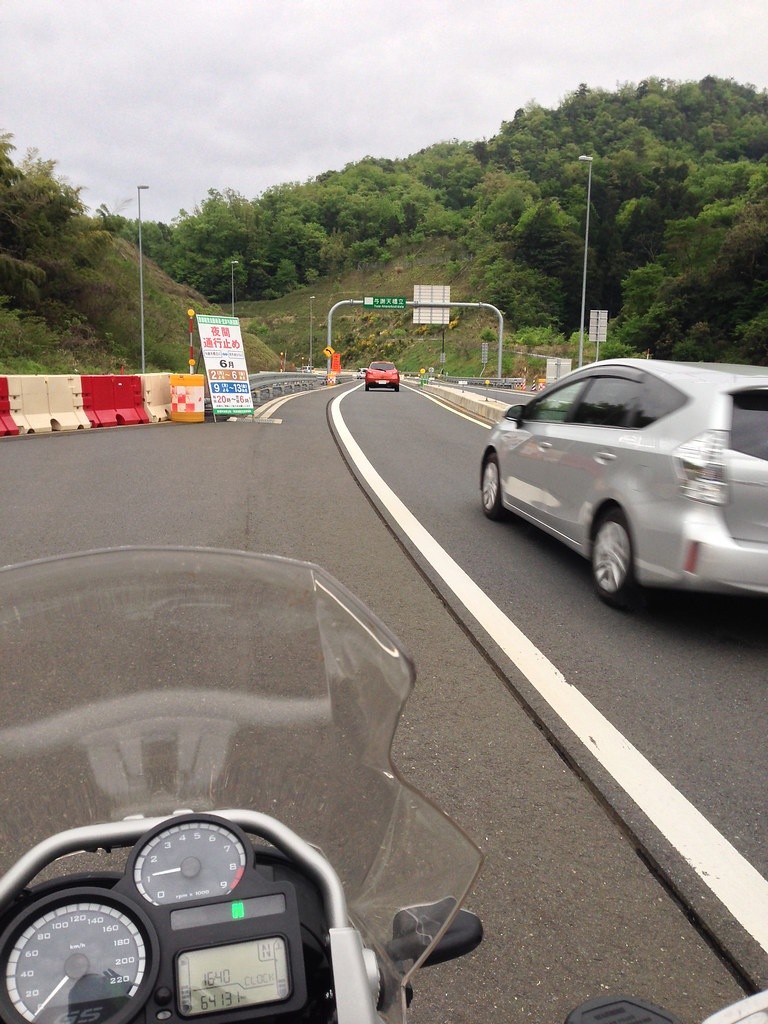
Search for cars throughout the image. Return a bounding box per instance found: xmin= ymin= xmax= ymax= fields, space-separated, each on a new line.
xmin=357 ymin=368 xmax=368 ymax=379
xmin=479 ymin=358 xmax=768 ymax=613
xmin=363 ymin=362 xmax=401 ymax=392
xmin=301 ymin=366 xmax=314 ymax=372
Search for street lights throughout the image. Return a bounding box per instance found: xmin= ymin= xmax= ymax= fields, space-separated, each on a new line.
xmin=578 ymin=156 xmax=593 ymax=367
xmin=231 ymin=261 xmax=239 ymax=316
xmin=310 ymin=296 xmax=316 ymax=373
xmin=137 ymin=185 xmax=149 ymax=374
